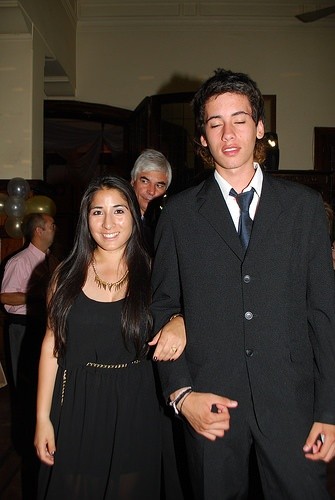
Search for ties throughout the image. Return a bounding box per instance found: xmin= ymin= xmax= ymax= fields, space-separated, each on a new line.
xmin=229 ymin=188 xmax=256 ymax=252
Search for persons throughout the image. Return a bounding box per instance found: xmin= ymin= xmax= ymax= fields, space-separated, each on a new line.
xmin=0 ymin=148 xmax=188 ymax=500
xmin=149 ymin=67 xmax=335 ymax=500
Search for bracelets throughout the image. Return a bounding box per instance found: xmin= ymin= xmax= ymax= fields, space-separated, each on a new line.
xmin=170 ymin=387 xmax=192 ymax=416
xmin=170 ymin=313 xmax=183 ymax=319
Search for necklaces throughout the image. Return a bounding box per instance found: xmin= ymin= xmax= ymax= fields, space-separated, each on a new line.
xmin=89 ymin=254 xmax=129 ymax=291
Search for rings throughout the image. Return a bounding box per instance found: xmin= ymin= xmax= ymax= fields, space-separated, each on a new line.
xmin=172 ymin=347 xmax=178 ymax=352
